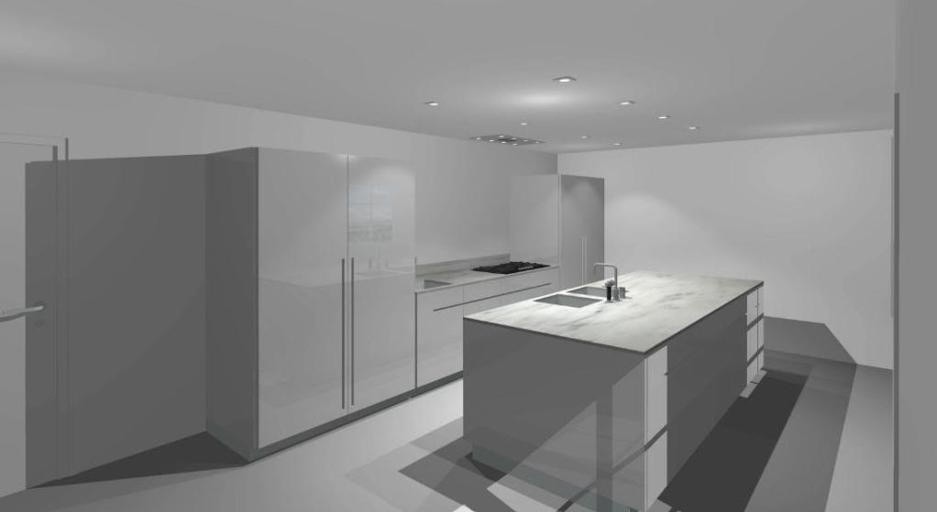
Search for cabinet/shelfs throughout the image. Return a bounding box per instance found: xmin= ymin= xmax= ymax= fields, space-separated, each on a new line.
xmin=414 ymin=252 xmax=557 ymax=396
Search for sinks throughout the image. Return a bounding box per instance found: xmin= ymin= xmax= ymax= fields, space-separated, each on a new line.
xmin=568 ymin=286 xmax=629 ymax=299
xmin=423 ymin=280 xmax=453 ymax=290
xmin=533 ymin=293 xmax=601 ymax=307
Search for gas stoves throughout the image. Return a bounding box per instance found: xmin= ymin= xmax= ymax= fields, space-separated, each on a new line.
xmin=469 ymin=261 xmax=552 ymax=276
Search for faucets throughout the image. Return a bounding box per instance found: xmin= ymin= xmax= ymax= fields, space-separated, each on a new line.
xmin=592 ymin=262 xmax=621 ymax=302
